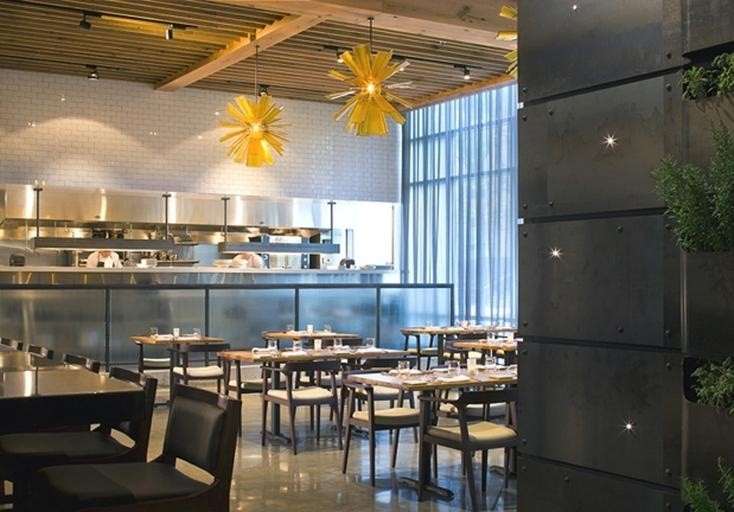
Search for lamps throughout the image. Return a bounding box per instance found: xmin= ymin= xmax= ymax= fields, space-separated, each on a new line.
xmin=323 ymin=18 xmax=418 ymax=140
xmin=218 ymin=44 xmax=294 ymax=170
xmin=165 ymin=24 xmax=175 ymax=41
xmin=87 ymin=65 xmax=100 ymax=81
xmin=462 ymin=66 xmax=471 ymax=80
xmin=80 ymin=13 xmax=92 ymax=31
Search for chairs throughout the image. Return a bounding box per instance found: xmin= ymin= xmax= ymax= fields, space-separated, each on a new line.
xmin=1 ymin=326 xmax=520 ymax=512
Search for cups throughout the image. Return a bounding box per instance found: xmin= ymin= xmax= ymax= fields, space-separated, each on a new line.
xmin=268 ymin=339 xmax=278 ymax=350
xmin=398 ymin=356 xmax=499 ymax=379
xmin=149 ymin=327 xmax=159 ymax=341
xmin=422 ymin=319 xmax=515 ymax=343
xmin=284 ymin=322 xmax=347 ymax=350
xmin=193 ymin=328 xmax=201 ymax=339
